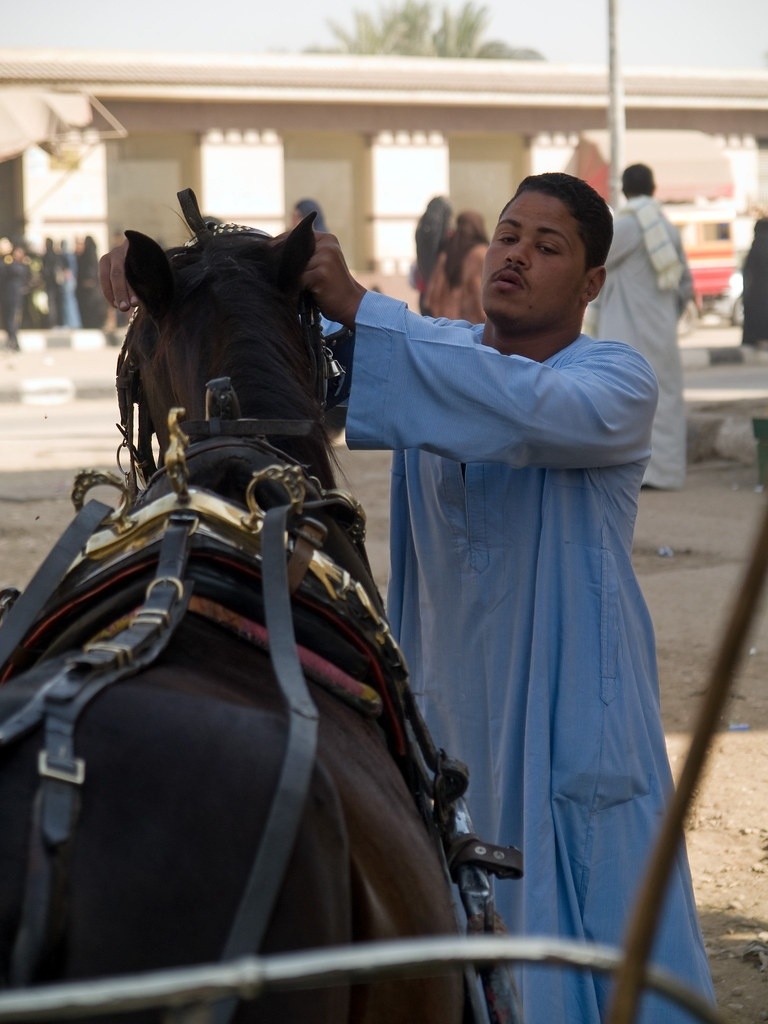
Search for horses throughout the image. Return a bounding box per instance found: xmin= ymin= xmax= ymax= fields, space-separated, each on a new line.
xmin=1 ymin=210 xmax=471 ymax=1024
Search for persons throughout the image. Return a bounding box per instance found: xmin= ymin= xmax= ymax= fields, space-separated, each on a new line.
xmin=288 ymin=173 xmax=714 ymax=1024
xmin=0 ymin=164 xmax=768 ymax=488
